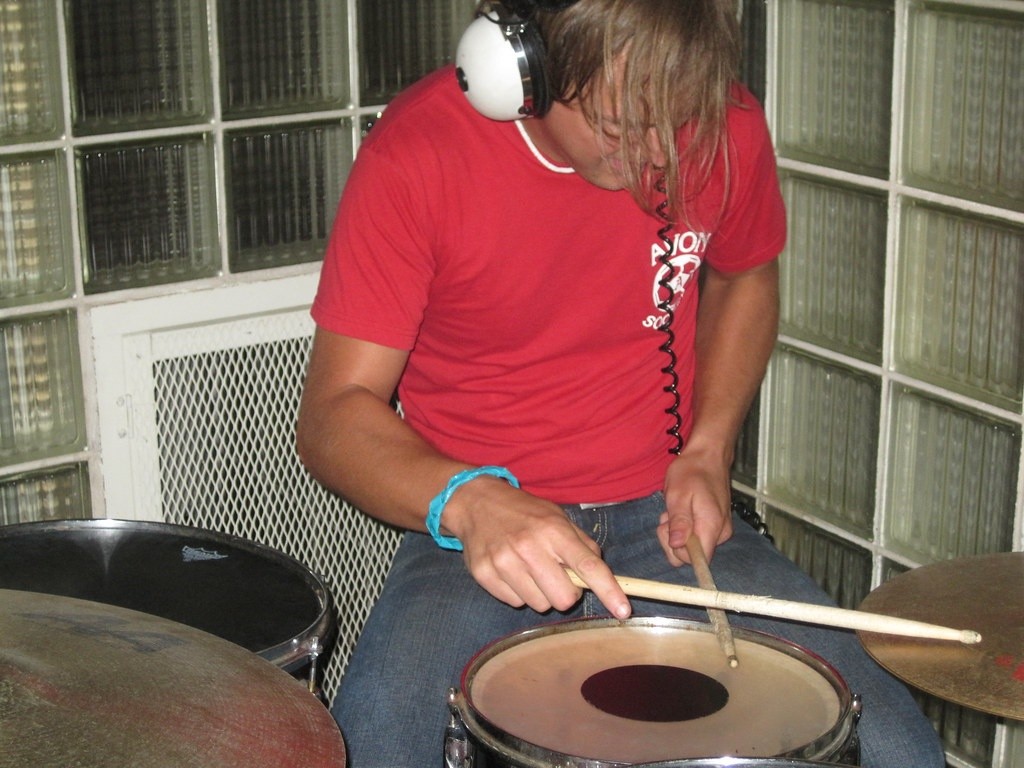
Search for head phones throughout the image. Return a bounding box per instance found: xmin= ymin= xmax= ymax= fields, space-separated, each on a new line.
xmin=455 ymin=0 xmax=553 ymax=120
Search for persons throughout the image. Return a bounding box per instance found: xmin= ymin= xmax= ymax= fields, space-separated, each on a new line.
xmin=296 ymin=1 xmax=946 ymax=768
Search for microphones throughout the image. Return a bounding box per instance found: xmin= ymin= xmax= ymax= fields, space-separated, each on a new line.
xmin=514 ymin=120 xmax=575 ymax=173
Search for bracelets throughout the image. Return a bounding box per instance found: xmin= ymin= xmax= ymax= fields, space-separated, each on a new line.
xmin=424 ymin=466 xmax=519 ymax=551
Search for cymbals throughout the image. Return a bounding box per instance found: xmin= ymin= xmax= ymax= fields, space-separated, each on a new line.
xmin=860 ymin=551 xmax=1022 ymax=725
xmin=0 ymin=589 xmax=348 ymax=768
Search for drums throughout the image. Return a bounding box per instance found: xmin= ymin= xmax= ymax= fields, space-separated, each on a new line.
xmin=442 ymin=616 xmax=863 ymax=768
xmin=2 ymin=519 xmax=332 ymax=670
